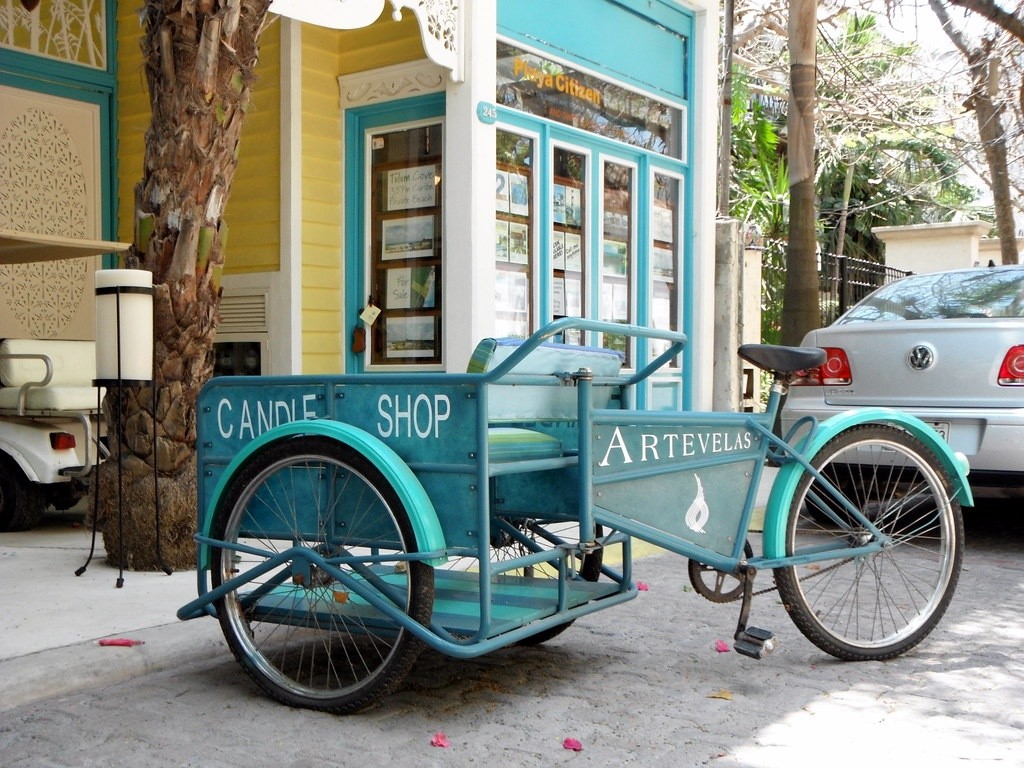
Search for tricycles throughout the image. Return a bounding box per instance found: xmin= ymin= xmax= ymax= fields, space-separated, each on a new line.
xmin=175 ymin=316 xmax=976 ymax=713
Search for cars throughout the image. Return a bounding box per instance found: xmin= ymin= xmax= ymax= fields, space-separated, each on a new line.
xmin=777 ymin=265 xmax=1024 ymax=523
